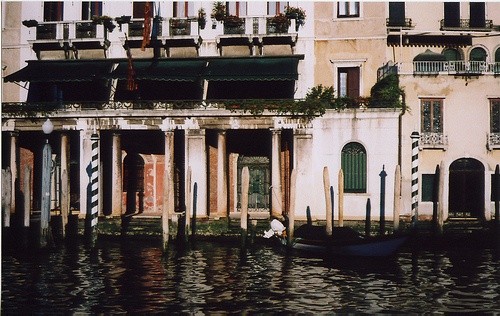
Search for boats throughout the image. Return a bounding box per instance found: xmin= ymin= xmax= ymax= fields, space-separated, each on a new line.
xmin=266 ymin=218 xmax=414 ymax=262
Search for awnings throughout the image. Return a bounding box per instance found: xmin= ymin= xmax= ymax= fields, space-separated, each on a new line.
xmin=108 ymin=61 xmax=206 ymax=83
xmin=7 ymin=60 xmax=114 ymax=86
xmin=203 ymin=56 xmax=300 ymax=82
